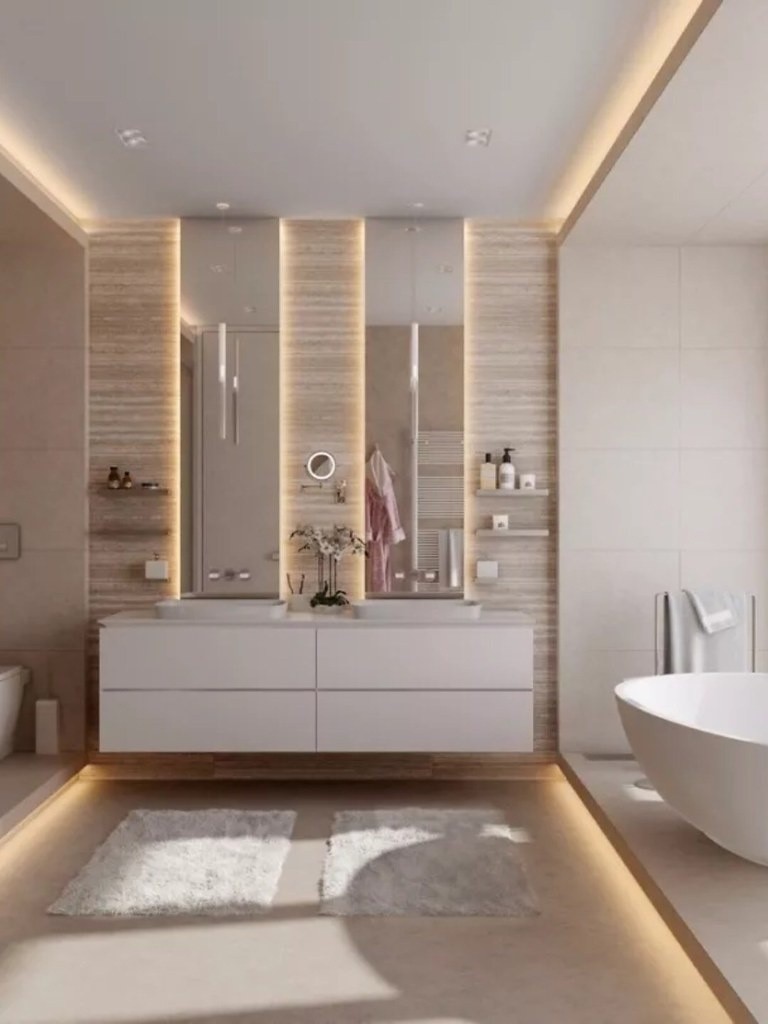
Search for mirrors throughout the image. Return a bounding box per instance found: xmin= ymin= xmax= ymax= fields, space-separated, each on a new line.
xmin=178 ymin=217 xmax=281 ymax=597
xmin=363 ymin=218 xmax=465 ymax=600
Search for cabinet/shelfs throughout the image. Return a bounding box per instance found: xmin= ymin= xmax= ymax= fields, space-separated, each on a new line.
xmin=316 ymin=625 xmax=534 ymax=753
xmin=476 ymin=487 xmax=550 ymax=536
xmin=95 ymin=627 xmax=317 ymax=752
xmin=97 ymin=486 xmax=170 ymax=536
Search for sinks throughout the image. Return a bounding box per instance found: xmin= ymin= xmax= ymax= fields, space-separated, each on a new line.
xmin=155 ymin=593 xmax=291 ymax=621
xmin=348 ymin=596 xmax=484 ymax=621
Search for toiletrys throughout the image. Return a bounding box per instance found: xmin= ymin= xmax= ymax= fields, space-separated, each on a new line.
xmin=475 ymin=550 xmax=500 ymax=580
xmin=478 ymin=452 xmax=499 ymax=491
xmin=491 ymin=513 xmax=511 ymax=529
xmin=519 ymin=474 xmax=538 ymax=490
xmin=143 ymin=550 xmax=169 ymax=582
xmin=498 ymin=446 xmax=517 ymax=489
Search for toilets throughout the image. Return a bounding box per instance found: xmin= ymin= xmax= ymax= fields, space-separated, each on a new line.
xmin=0 ymin=663 xmax=30 ymax=762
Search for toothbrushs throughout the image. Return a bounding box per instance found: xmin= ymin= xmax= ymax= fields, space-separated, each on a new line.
xmin=286 ymin=571 xmax=297 ymax=594
xmin=298 ymin=574 xmax=307 ymax=595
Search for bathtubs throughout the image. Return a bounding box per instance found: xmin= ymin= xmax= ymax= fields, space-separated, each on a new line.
xmin=611 ymin=665 xmax=768 ymax=868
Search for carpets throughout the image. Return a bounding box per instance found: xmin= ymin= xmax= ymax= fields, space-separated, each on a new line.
xmin=319 ymin=807 xmax=544 ymax=917
xmin=46 ymin=808 xmax=298 ymax=916
